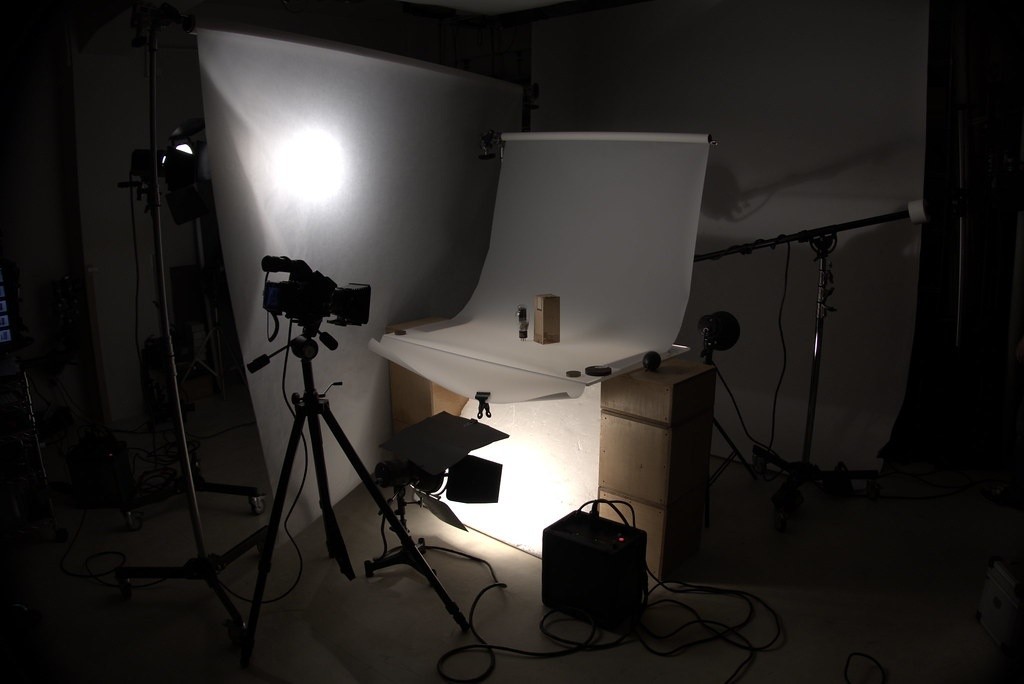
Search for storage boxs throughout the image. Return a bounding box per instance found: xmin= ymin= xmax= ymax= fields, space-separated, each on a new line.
xmin=534 ymin=294 xmax=561 ymax=343
xmin=975 ymin=553 xmax=1024 ymax=656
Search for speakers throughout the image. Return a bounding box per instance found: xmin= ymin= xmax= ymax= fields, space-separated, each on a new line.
xmin=540 ymin=509 xmax=647 ymax=639
xmin=65 ymin=434 xmax=138 ymax=510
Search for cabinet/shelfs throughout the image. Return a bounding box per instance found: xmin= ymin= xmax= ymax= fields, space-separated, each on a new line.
xmin=387 ymin=315 xmax=717 ymax=585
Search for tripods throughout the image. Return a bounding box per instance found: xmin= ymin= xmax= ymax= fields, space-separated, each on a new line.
xmin=115 ymin=30 xmax=471 ymax=666
xmin=699 ymin=346 xmax=760 ymax=533
